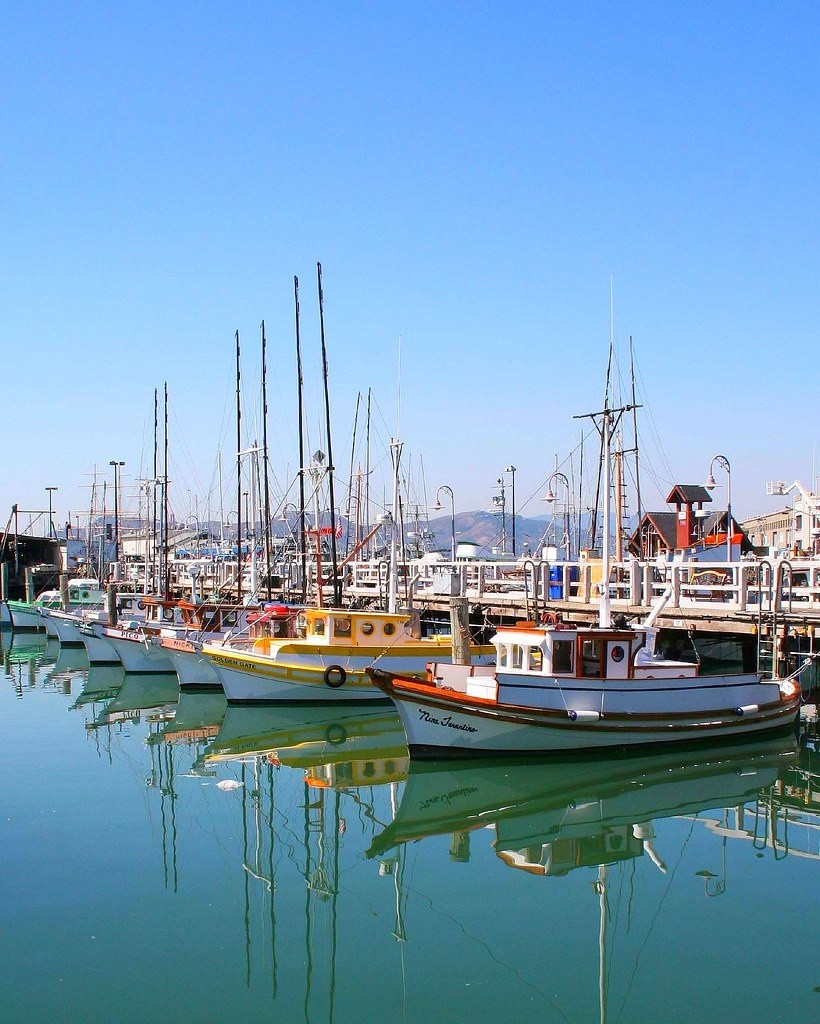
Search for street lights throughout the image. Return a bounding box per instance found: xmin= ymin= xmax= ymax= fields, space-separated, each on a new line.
xmin=540 ymin=473 xmax=570 ymax=563
xmin=278 ymin=503 xmax=298 ymax=584
xmin=341 ymin=496 xmax=359 ymax=562
xmin=45 ymin=487 xmax=59 ymax=537
xmin=183 ymin=515 xmax=199 ymax=559
xmin=506 ymin=465 xmax=516 ymax=557
xmin=701 ymin=454 xmax=733 ymax=587
xmin=429 ymin=485 xmax=456 ymax=562
xmin=243 ymin=492 xmax=249 ymax=553
xmin=110 ymin=462 xmax=125 ymax=561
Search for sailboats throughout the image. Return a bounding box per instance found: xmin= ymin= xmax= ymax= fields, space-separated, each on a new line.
xmin=3 ymin=632 xmax=790 ymax=1023
xmin=3 ymin=260 xmax=801 ymax=758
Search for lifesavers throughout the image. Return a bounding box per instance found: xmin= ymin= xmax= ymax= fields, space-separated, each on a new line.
xmin=324 ymin=663 xmax=347 ymax=689
xmin=324 ymin=723 xmax=347 ymax=745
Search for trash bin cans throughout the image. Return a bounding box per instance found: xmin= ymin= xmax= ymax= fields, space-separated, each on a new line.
xmin=549 ymin=565 xmax=579 ymax=599
